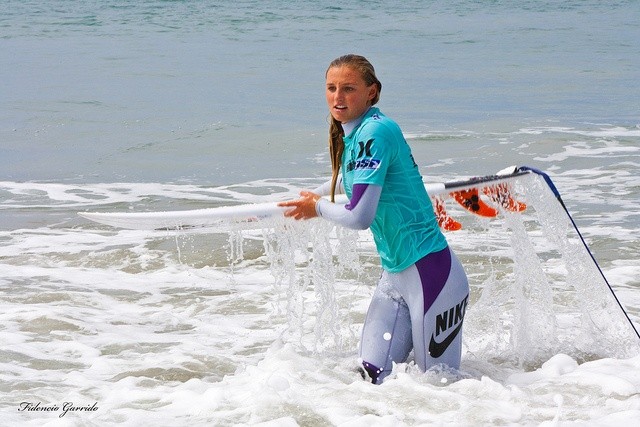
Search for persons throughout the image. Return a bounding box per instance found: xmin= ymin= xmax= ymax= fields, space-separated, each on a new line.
xmin=247 ymin=53 xmax=471 ymax=388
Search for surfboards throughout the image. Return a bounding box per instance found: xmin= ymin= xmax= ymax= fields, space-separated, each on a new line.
xmin=78 ymin=164 xmax=532 ymax=231
